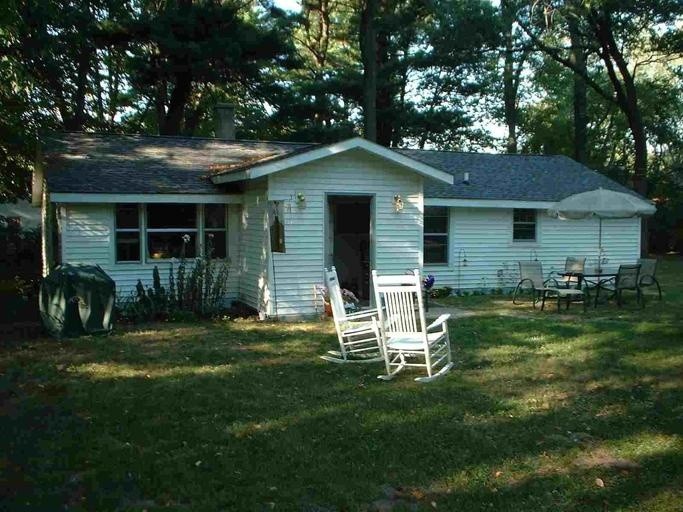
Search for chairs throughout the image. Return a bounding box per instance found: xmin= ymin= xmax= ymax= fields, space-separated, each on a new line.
xmin=511 ymin=256 xmax=663 ymax=314
xmin=319 ymin=269 xmax=452 ymax=386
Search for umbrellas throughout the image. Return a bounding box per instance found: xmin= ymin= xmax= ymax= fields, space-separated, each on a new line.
xmin=546 ymin=186 xmax=658 ymax=293
